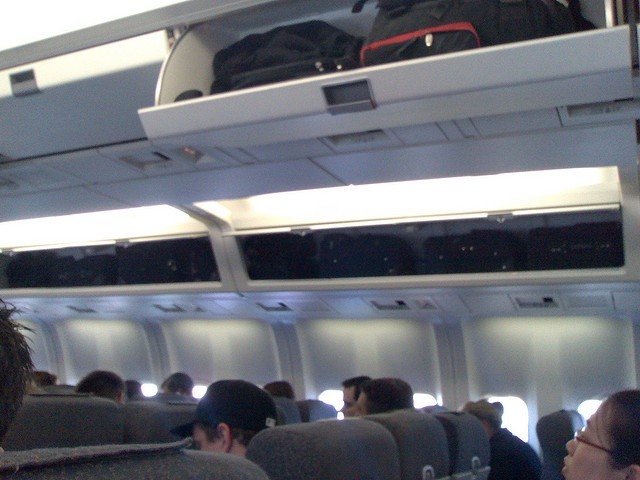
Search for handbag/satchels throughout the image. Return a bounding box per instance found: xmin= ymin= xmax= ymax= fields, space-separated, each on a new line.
xmin=211 ymin=20 xmax=367 ymax=94
xmin=352 ymin=0 xmax=598 ymax=67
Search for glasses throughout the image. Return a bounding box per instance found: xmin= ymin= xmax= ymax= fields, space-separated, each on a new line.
xmin=573 ymin=427 xmax=613 ymax=454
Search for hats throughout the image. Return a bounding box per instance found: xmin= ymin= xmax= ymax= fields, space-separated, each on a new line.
xmin=169 ymin=379 xmax=278 ymax=438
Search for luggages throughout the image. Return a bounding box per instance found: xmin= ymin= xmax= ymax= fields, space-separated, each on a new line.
xmin=527 ymin=221 xmax=625 ymax=270
xmin=6 ymin=253 xmax=77 ymax=288
xmin=242 ymin=233 xmax=320 ymax=280
xmin=54 ymin=255 xmax=117 ymax=286
xmin=321 ymin=234 xmax=417 ymax=278
xmin=421 ymin=229 xmax=527 ymax=274
xmin=112 ymin=236 xmax=216 ymax=285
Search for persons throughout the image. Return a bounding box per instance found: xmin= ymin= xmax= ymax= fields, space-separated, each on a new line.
xmin=263 ymin=380 xmax=295 ymax=399
xmin=347 ymin=379 xmax=412 ymax=416
xmin=170 ymin=378 xmax=277 ymax=457
xmin=559 ymin=388 xmax=640 ymax=479
xmin=458 ymin=398 xmax=542 ymax=480
xmin=160 ymin=372 xmax=199 ymax=400
xmin=33 ymin=371 xmax=60 ymax=386
xmin=339 ymin=376 xmax=372 ymax=418
xmin=123 ymin=380 xmax=143 ymax=392
xmin=1 ymin=302 xmax=35 ymax=479
xmin=77 ymin=372 xmax=126 ymax=403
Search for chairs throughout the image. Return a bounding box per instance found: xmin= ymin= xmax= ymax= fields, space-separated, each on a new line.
xmin=0 ymin=436 xmax=270 ymax=479
xmin=432 ymin=410 xmax=492 ymax=480
xmin=0 ymin=394 xmax=125 ymax=451
xmin=123 ymin=400 xmax=198 ymax=445
xmin=362 ymin=408 xmax=453 ymax=480
xmin=154 ymin=392 xmax=201 ymax=404
xmin=271 ymin=395 xmax=303 ymax=424
xmin=535 ymin=409 xmax=588 ymax=480
xmin=297 ymin=398 xmax=338 ymax=422
xmin=244 ymin=416 xmax=402 ymax=480
